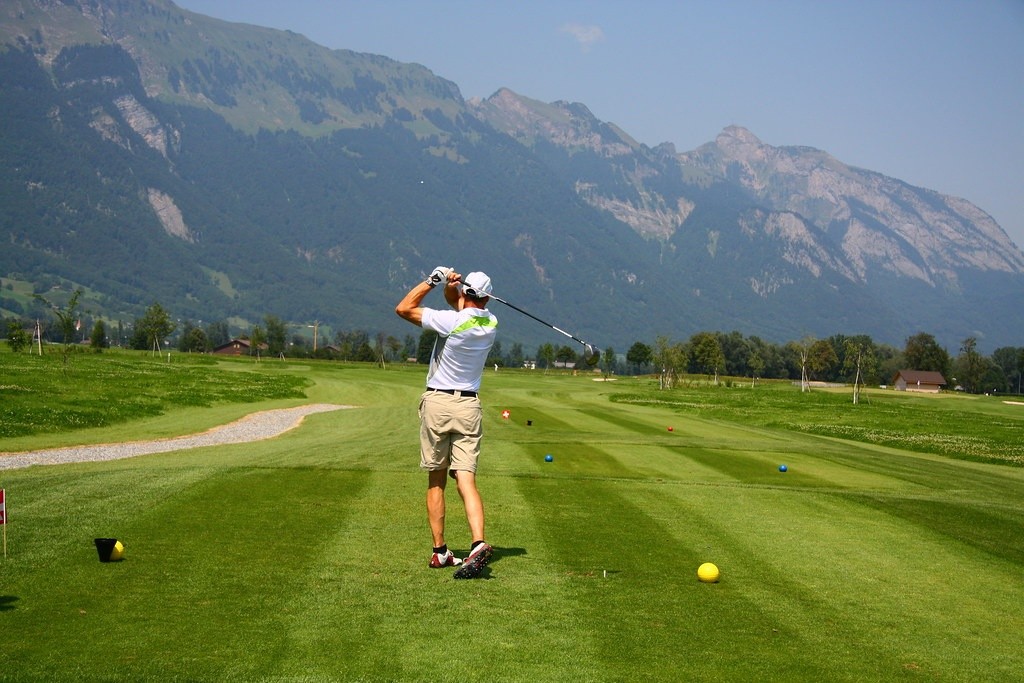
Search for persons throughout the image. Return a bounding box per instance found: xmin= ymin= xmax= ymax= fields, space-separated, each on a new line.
xmin=395 ymin=266 xmax=498 ymax=578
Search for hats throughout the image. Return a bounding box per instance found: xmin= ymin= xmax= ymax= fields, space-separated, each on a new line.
xmin=463 ymin=272 xmax=493 ymax=298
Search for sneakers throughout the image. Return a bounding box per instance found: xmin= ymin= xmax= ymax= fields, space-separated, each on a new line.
xmin=453 ymin=542 xmax=493 ymax=579
xmin=429 ymin=549 xmax=462 ymax=568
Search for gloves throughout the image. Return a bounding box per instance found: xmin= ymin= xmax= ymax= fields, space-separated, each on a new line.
xmin=426 ymin=266 xmax=454 ymax=288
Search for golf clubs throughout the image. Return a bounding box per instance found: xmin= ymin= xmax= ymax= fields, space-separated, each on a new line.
xmin=456 ymin=278 xmax=601 ymax=365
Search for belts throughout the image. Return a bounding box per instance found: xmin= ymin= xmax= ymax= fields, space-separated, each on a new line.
xmin=427 ymin=387 xmax=478 ymax=398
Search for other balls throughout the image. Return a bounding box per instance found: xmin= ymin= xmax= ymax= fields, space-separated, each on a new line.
xmin=668 ymin=427 xmax=673 ymax=431
xmin=545 ymin=455 xmax=553 ymax=462
xmin=779 ymin=465 xmax=787 ymax=472
xmin=110 ymin=541 xmax=124 ymax=560
xmin=697 ymin=563 xmax=720 ymax=582
